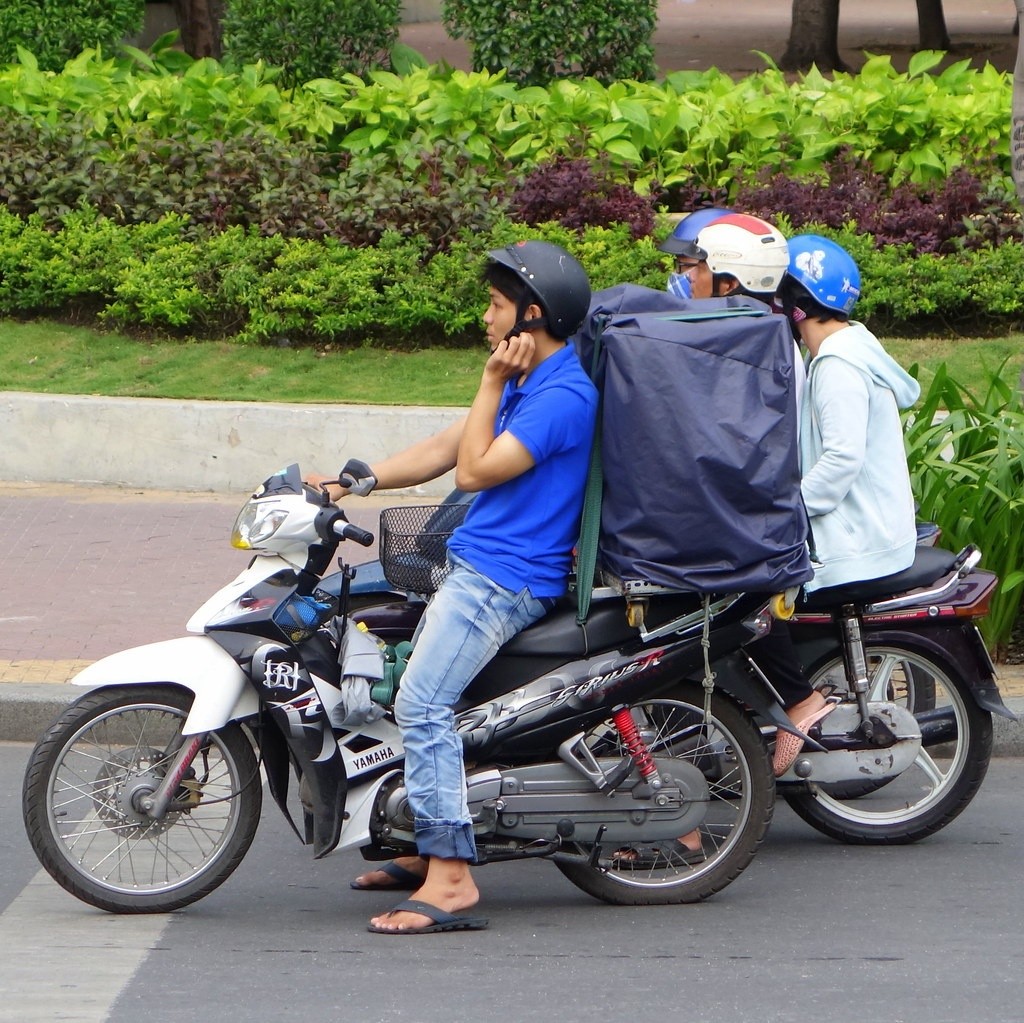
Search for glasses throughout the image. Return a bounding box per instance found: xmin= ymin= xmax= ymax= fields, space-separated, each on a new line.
xmin=673 ymin=258 xmax=698 ymax=274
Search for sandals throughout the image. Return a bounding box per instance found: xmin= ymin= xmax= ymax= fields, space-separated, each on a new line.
xmin=771 ymin=701 xmax=838 ymax=779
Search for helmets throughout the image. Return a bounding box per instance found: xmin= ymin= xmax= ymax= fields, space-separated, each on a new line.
xmin=656 ymin=207 xmax=861 ymax=316
xmin=485 ymin=239 xmax=591 ymax=338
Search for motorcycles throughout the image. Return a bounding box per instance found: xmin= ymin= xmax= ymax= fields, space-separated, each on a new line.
xmin=22 ymin=460 xmax=828 ymax=917
xmin=282 ymin=487 xmax=1019 ymax=847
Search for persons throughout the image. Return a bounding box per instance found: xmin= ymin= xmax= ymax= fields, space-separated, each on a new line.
xmin=612 ymin=208 xmax=921 ymax=869
xmin=304 ymin=238 xmax=597 ymax=936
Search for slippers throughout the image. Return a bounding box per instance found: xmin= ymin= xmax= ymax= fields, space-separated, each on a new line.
xmin=349 ymin=862 xmax=426 ymax=889
xmin=367 ymin=901 xmax=491 ymax=935
xmin=613 ymin=838 xmax=708 ymax=868
